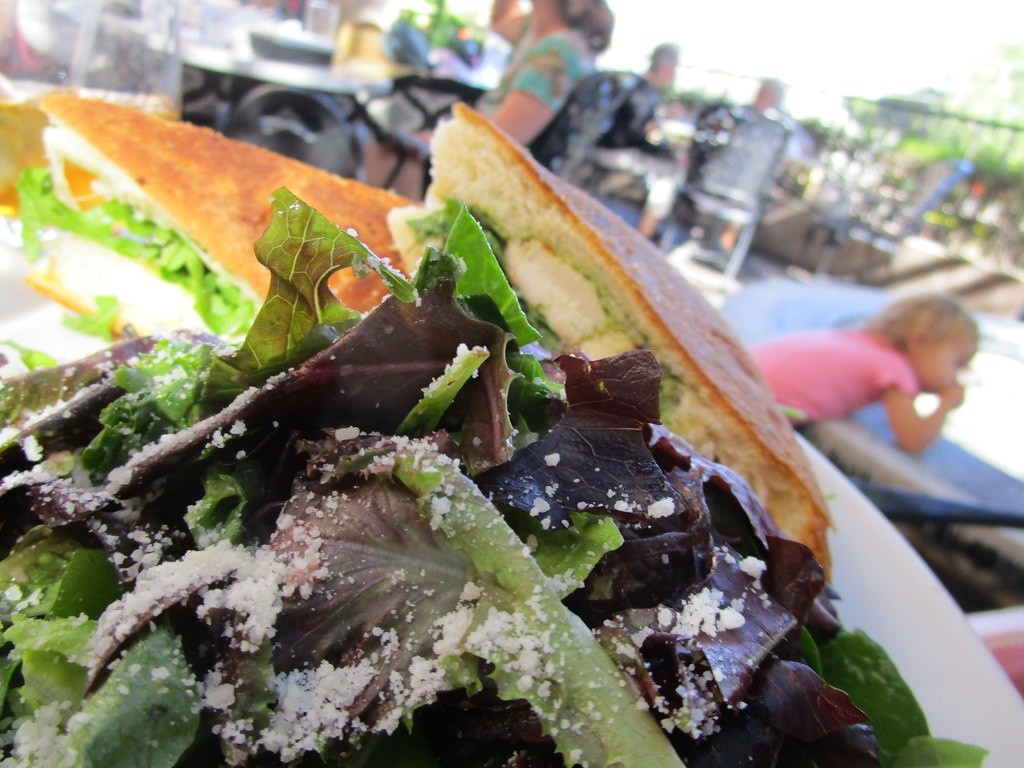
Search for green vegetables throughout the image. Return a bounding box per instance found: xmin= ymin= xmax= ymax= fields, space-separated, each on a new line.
xmin=0 ymin=186 xmax=986 ymax=768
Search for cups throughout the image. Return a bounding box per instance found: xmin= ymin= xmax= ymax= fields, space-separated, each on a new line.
xmin=1 ymin=0 xmax=186 ymax=121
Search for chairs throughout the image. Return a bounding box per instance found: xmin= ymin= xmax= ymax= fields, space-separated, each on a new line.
xmin=175 ymin=44 xmax=793 ymax=279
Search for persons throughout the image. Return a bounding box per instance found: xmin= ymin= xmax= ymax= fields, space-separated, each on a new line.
xmin=356 ymin=0 xmax=789 ymax=253
xmin=750 ymin=294 xmax=981 ymax=458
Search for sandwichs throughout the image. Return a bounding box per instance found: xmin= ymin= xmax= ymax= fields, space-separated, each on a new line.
xmin=3 ymin=89 xmax=828 ymax=572
